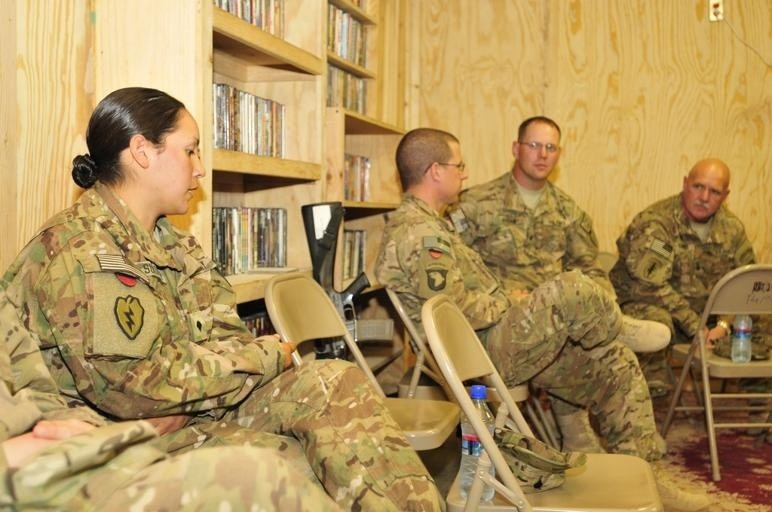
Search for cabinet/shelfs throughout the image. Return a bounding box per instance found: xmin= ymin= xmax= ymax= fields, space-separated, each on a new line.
xmin=92 ymin=0 xmax=410 ymax=397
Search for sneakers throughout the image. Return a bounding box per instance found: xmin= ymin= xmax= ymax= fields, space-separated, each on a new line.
xmin=649 ymin=458 xmax=714 ymax=511
xmin=616 ymin=314 xmax=670 ymax=353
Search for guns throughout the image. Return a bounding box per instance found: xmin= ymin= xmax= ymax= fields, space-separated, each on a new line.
xmin=300 ymin=202 xmax=371 ymax=362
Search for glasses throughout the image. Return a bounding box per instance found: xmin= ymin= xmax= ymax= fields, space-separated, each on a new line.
xmin=519 ymin=141 xmax=556 ymax=153
xmin=424 ymin=160 xmax=465 ymax=173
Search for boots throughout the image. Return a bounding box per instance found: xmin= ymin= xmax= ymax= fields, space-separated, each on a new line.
xmin=555 ymin=409 xmax=609 ymax=454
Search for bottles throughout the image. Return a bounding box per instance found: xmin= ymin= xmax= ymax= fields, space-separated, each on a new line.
xmin=731 ymin=314 xmax=753 ymax=364
xmin=458 ymin=385 xmax=496 ymax=503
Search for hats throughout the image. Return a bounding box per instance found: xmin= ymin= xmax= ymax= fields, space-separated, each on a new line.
xmin=495 ymin=424 xmax=566 ymax=493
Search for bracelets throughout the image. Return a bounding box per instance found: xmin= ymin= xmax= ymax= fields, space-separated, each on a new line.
xmin=716 ymin=319 xmax=731 ymax=335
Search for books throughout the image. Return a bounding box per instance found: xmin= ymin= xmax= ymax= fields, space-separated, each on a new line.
xmin=341 ymin=153 xmax=373 ymax=282
xmin=212 ymin=0 xmax=287 ymax=42
xmin=327 ymin=1 xmax=370 ymax=118
xmin=235 ymin=312 xmax=276 ymax=340
xmin=209 ymin=203 xmax=290 ymax=277
xmin=213 ymin=81 xmax=289 ymax=160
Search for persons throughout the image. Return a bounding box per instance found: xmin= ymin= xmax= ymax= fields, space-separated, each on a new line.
xmin=0 ymin=291 xmax=346 ymax=512
xmin=604 ymin=156 xmax=772 ymax=442
xmin=441 ymin=114 xmax=620 ymax=452
xmin=372 ymin=127 xmax=714 ymax=511
xmin=1 ymin=83 xmax=453 ymax=512
